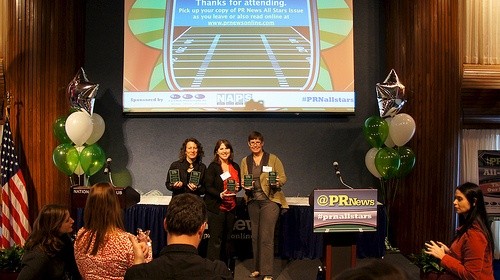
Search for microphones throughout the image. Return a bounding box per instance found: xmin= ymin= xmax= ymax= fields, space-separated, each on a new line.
xmin=104 ymin=157 xmax=112 ymax=175
xmin=333 ymin=162 xmax=341 ymax=178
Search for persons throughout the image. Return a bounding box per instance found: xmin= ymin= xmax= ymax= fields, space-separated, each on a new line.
xmin=423 ymin=182 xmax=496 ymax=280
xmin=336 ymin=263 xmax=410 ymax=280
xmin=15 ymin=204 xmax=83 ymax=280
xmin=123 ymin=193 xmax=231 ymax=280
xmin=240 ymin=131 xmax=286 ymax=280
xmin=203 ymin=139 xmax=242 ymax=274
xmin=165 ymin=137 xmax=207 ymax=198
xmin=74 ymin=182 xmax=138 ymax=280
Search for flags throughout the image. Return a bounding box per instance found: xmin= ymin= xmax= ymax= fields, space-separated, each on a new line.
xmin=0 ymin=118 xmax=31 ymax=250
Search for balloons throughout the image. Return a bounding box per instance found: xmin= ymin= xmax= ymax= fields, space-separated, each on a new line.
xmin=66 ymin=68 xmax=99 ymax=110
xmin=52 ymin=106 xmax=105 ymax=178
xmin=361 ymin=113 xmax=416 ymax=180
xmin=375 ymin=69 xmax=406 ymax=116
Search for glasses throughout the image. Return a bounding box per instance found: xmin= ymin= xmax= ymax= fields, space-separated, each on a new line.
xmin=249 ymin=142 xmax=262 ymax=147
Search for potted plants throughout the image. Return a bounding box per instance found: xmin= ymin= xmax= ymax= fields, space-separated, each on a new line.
xmin=0 ymin=242 xmax=24 ymax=280
xmin=412 ymin=242 xmax=445 ymax=280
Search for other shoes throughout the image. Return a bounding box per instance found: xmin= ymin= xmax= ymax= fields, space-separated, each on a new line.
xmin=262 ymin=276 xmax=273 ymax=280
xmin=249 ymin=271 xmax=259 ymax=278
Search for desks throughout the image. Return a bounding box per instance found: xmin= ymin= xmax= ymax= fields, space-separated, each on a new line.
xmin=73 ymin=192 xmax=385 ymax=263
